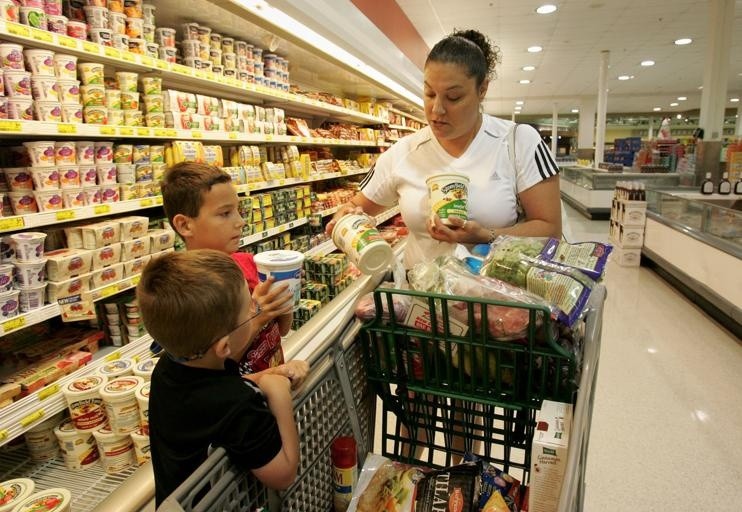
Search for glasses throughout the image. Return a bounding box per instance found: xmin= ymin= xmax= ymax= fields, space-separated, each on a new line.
xmin=199 ymin=299 xmax=262 ymax=361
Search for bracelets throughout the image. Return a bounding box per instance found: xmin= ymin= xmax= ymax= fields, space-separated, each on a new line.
xmin=488 ymin=229 xmax=496 ymax=244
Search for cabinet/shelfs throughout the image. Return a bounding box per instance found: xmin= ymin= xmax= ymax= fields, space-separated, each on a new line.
xmin=558 ymin=164 xmax=680 ymax=220
xmin=0 ymin=0 xmax=445 ymax=511
xmin=593 ymin=107 xmax=739 ymax=163
xmin=643 ymin=186 xmax=742 ymax=343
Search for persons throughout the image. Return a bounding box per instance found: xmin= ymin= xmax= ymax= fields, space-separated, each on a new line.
xmin=325 ymin=26 xmax=562 ymax=464
xmin=161 ymin=161 xmax=295 ymax=375
xmin=136 ymin=248 xmax=312 ymax=511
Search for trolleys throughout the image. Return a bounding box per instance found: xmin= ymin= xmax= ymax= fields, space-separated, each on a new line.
xmin=159 ymin=239 xmax=607 ymax=512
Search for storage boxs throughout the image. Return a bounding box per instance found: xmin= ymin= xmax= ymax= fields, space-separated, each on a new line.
xmin=608 ymin=198 xmax=648 ymax=267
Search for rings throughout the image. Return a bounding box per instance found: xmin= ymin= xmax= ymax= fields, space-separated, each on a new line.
xmin=462 ymin=219 xmax=466 ymax=228
